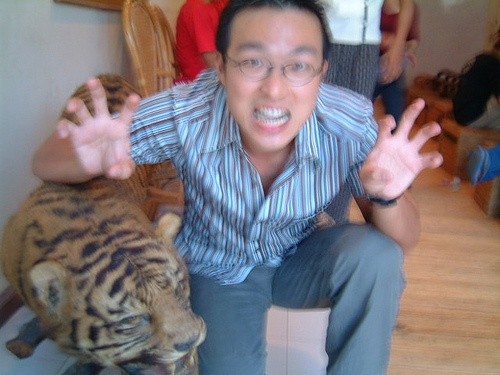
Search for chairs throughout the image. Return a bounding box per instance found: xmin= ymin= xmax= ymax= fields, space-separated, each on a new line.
xmin=122 ymin=0 xmax=187 ymax=219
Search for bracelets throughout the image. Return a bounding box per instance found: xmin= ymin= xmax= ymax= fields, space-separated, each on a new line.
xmin=364 ymin=191 xmax=406 ymax=207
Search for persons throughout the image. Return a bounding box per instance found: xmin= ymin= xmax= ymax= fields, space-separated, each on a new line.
xmin=32 ymin=0 xmax=444 ymax=375
xmin=174 ymin=1 xmax=500 ymax=181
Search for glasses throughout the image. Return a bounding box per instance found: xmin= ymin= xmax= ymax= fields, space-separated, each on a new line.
xmin=225 ymin=55 xmax=323 ymax=83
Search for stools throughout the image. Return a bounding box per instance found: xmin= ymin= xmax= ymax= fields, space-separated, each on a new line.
xmin=439 ymin=118 xmax=496 ymax=175
